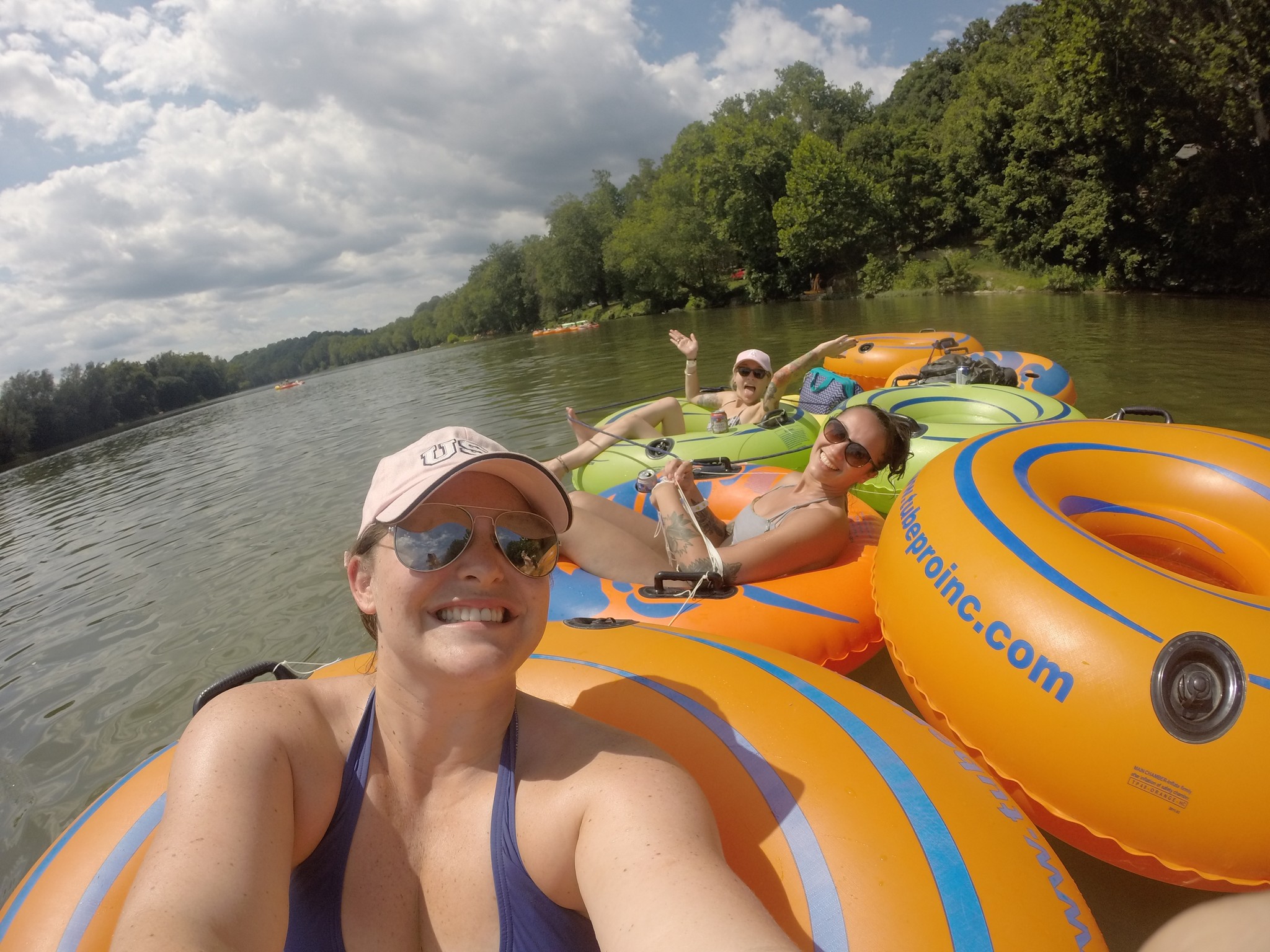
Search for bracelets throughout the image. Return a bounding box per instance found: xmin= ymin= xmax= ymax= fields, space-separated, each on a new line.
xmin=684 ymin=359 xmax=698 ymax=375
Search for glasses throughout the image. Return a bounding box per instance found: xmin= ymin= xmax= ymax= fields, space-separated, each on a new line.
xmin=737 ymin=366 xmax=769 ymax=379
xmin=387 ymin=502 xmax=562 ymax=578
xmin=823 ymin=416 xmax=878 ymax=472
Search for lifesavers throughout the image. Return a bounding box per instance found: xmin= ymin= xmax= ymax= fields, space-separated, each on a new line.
xmin=872 ymin=422 xmax=1270 ymax=893
xmin=570 ymin=388 xmax=820 ymax=497
xmin=817 ymin=383 xmax=1092 ymax=519
xmin=819 ymin=329 xmax=985 ymax=388
xmin=549 ymin=455 xmax=887 ymax=675
xmin=884 ymin=348 xmax=1075 ymax=406
xmin=0 ymin=614 xmax=1115 ymax=952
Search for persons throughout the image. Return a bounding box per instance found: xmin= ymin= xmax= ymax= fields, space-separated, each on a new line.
xmin=556 ymin=403 xmax=914 ymax=590
xmin=541 ymin=329 xmax=858 ymax=479
xmin=105 ymin=426 xmax=804 ymax=952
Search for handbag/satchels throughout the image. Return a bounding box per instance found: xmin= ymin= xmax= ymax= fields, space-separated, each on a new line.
xmin=798 ymin=367 xmax=865 ymax=415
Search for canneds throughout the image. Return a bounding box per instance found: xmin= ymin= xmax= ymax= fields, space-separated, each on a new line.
xmin=637 ymin=469 xmax=657 ymax=493
xmin=710 ymin=410 xmax=729 ymax=434
xmin=956 ymin=365 xmax=971 ymax=385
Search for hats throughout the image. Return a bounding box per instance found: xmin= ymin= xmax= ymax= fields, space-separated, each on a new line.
xmin=357 ymin=427 xmax=574 ymax=541
xmin=733 ymin=349 xmax=773 ymax=377
